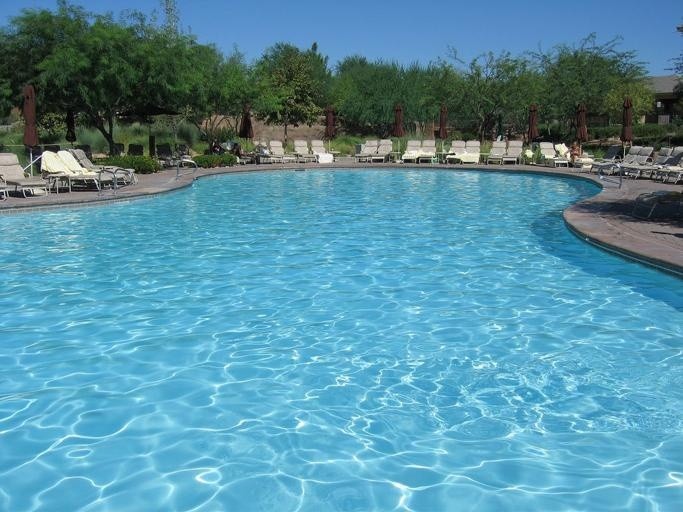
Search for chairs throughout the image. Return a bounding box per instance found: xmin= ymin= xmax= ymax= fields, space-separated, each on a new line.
xmin=0 ymin=141 xmax=142 ymax=199
xmin=589 ymin=145 xmax=683 ymax=185
xmin=154 ymin=141 xmax=192 ymax=168
xmin=486 ymin=140 xmax=524 ymax=166
xmin=358 ymin=139 xmax=481 ymax=165
xmin=213 ymin=140 xmax=332 ymax=168
xmin=539 ymin=142 xmax=594 ymax=167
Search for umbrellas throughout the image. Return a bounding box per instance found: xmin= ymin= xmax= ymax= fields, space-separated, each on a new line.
xmin=324 ymin=103 xmax=335 ymax=154
xmin=65 ymin=108 xmax=76 ymax=148
xmin=238 ymin=98 xmax=254 ymax=149
xmin=115 ymin=102 xmax=182 ymax=137
xmin=21 ymin=84 xmax=45 ymax=195
xmin=528 ymin=102 xmax=539 ymax=165
xmin=619 ymin=96 xmax=632 ymax=158
xmin=438 ymin=103 xmax=448 ymax=163
xmin=391 ymin=103 xmax=405 ymax=163
xmin=575 ymin=103 xmax=587 ymax=158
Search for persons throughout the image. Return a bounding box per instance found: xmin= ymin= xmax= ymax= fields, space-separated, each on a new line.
xmin=563 ymin=140 xmax=583 ymax=164
xmin=232 ymin=142 xmax=251 ymax=160
xmin=212 ymin=142 xmax=225 ymax=155
